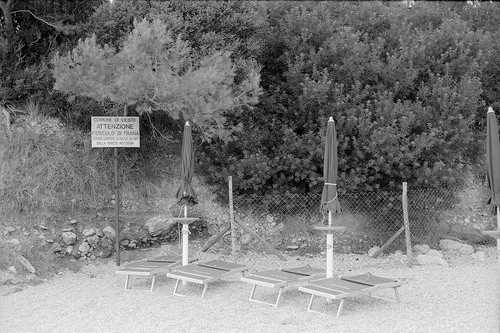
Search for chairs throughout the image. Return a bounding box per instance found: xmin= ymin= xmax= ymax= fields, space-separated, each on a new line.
xmin=167 ymin=260 xmax=249 ymax=298
xmin=240 ymin=265 xmax=338 ymax=307
xmin=299 ymin=272 xmax=402 ymax=320
xmin=116 ymin=256 xmax=199 ymax=293
